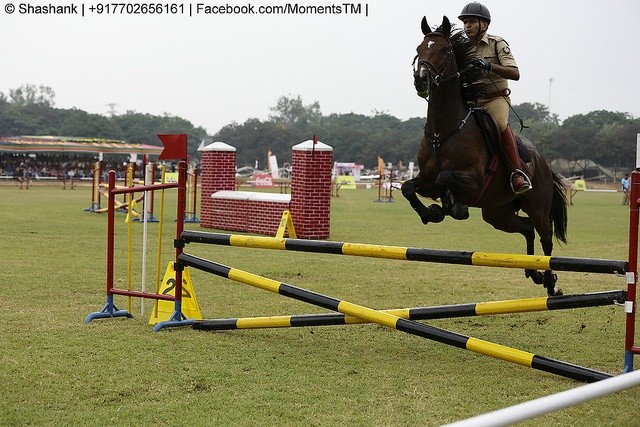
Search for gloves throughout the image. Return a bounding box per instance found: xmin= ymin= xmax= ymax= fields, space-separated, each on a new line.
xmin=467 ymin=56 xmax=492 ymax=71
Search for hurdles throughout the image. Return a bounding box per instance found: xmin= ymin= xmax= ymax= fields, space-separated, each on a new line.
xmin=152 ymin=162 xmax=640 ymax=388
xmin=569 ymin=189 xmax=631 ymax=207
xmin=375 ymin=169 xmax=394 ymax=203
xmin=200 ymin=141 xmax=331 ymax=239
xmin=84 ymin=160 xmax=199 ymax=222
xmin=85 ymin=163 xmax=189 ymax=333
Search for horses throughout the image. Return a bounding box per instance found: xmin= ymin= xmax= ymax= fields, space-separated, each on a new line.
xmin=399 ymin=15 xmax=570 ymax=298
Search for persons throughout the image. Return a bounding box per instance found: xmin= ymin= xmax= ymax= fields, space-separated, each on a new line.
xmin=1 ymin=153 xmax=128 ymax=180
xmin=349 ymin=170 xmax=354 ymax=176
xmin=620 ymin=172 xmax=630 ymax=207
xmin=339 ymin=169 xmax=343 ymax=175
xmin=458 ymin=2 xmax=530 ymax=192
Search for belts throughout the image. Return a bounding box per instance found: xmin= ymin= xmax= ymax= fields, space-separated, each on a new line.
xmin=479 ymin=90 xmax=508 ymax=98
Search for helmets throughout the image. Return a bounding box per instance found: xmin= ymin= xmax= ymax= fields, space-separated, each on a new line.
xmin=458 ymin=1 xmax=491 ymax=24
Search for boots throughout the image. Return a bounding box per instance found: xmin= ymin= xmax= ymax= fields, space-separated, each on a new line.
xmin=500 ymin=124 xmax=530 ymax=192
